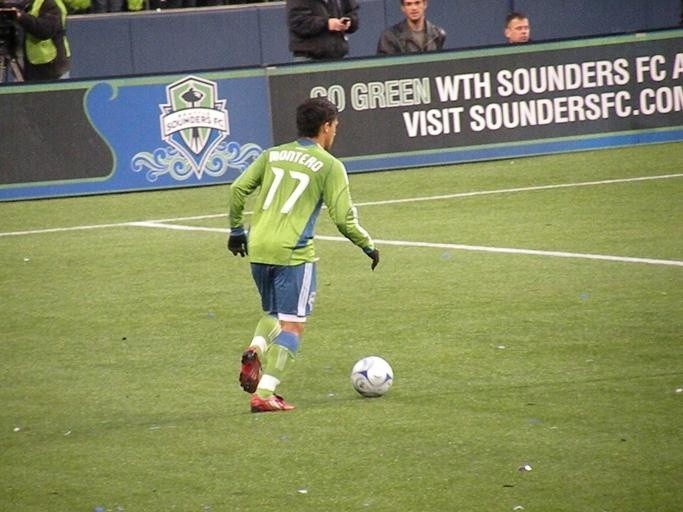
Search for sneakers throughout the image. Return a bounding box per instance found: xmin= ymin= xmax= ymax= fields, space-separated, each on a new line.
xmin=250 ymin=391 xmax=294 ymax=413
xmin=239 ymin=348 xmax=261 ymax=393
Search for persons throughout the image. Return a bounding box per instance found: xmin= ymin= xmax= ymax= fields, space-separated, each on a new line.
xmin=375 ymin=0 xmax=447 ymax=59
xmin=227 ymin=96 xmax=379 ymax=412
xmin=284 ymin=0 xmax=359 ymax=67
xmin=5 ymin=0 xmax=71 ymax=83
xmin=502 ymin=13 xmax=531 ymax=45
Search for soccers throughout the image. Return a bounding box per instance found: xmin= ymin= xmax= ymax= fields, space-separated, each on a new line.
xmin=350 ymin=355 xmax=392 ymax=397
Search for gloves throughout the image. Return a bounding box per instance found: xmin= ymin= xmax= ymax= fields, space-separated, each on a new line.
xmin=362 ymin=245 xmax=380 ymax=270
xmin=228 ymin=225 xmax=248 ymax=257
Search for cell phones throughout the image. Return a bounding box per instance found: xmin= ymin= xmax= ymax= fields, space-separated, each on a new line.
xmin=343 ymin=19 xmax=349 ymax=24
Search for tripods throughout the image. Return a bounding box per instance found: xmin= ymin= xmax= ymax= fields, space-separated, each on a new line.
xmin=0 ymin=55 xmax=24 ymax=82
